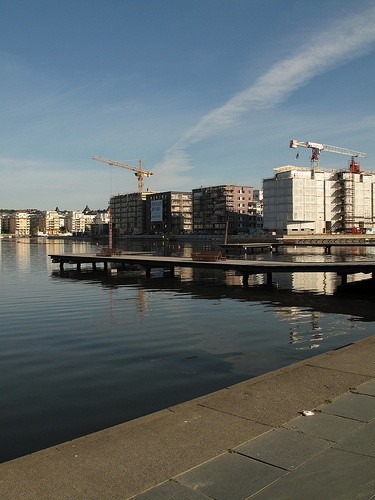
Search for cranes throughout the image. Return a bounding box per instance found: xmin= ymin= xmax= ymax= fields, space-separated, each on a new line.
xmin=91 ymin=154 xmax=153 ymax=192
xmin=290 ymin=139 xmax=366 ymax=172
